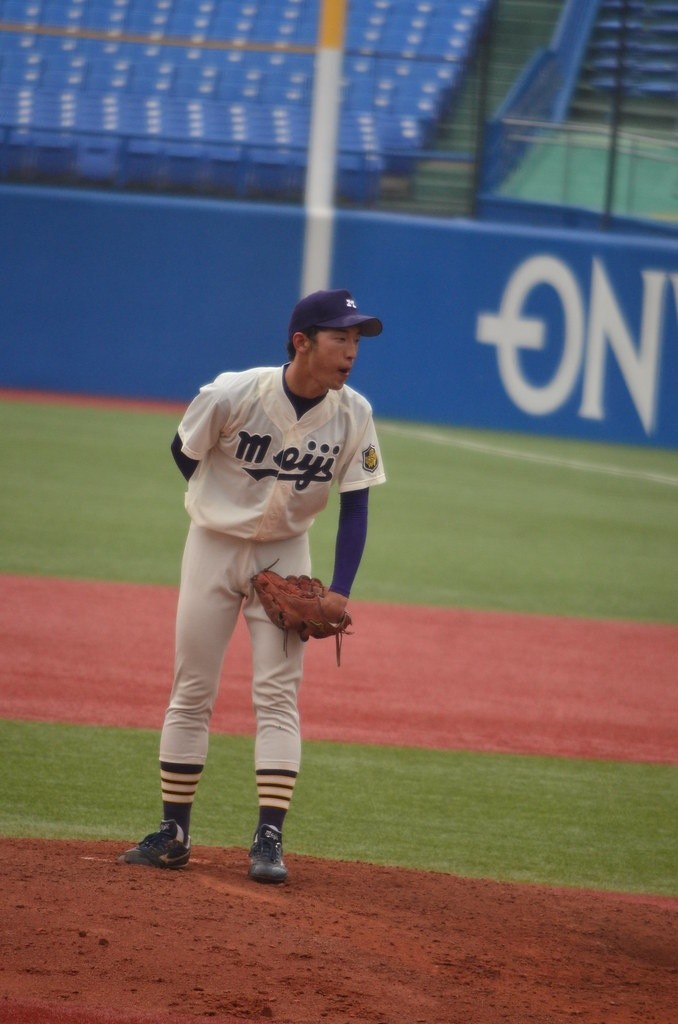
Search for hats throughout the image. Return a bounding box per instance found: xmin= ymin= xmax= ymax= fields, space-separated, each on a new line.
xmin=289 ymin=290 xmax=383 ymax=337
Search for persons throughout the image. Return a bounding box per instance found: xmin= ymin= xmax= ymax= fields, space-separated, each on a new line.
xmin=117 ymin=289 xmax=388 ymax=885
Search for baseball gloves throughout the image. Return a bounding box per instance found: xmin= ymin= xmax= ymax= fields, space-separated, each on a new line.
xmin=247 ymin=556 xmax=355 ymax=669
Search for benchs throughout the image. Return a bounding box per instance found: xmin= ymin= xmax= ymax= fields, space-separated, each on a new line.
xmin=0 ymin=1 xmax=678 ymax=206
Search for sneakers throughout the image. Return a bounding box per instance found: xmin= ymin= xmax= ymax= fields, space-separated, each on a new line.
xmin=118 ymin=819 xmax=191 ymax=869
xmin=249 ymin=825 xmax=288 ymax=885
